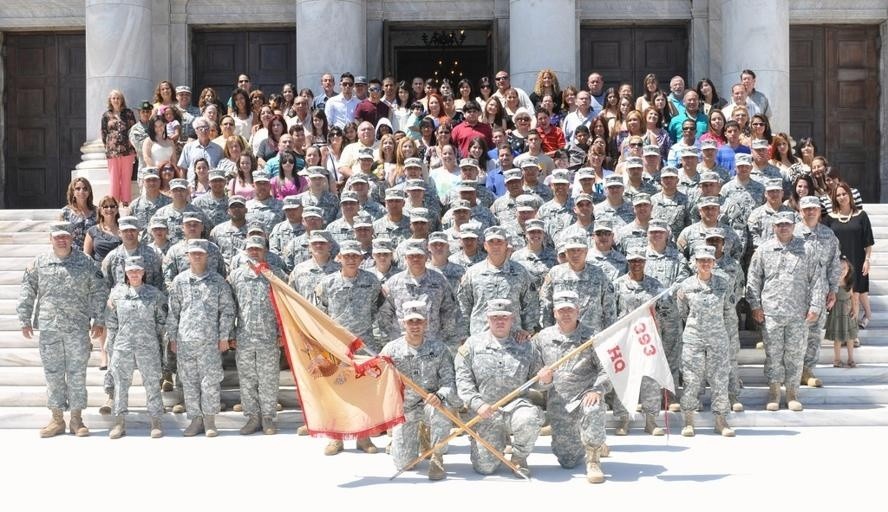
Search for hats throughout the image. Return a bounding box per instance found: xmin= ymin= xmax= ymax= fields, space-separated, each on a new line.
xmin=800 ymin=195 xmax=821 ymax=209
xmin=118 ymin=139 xmax=725 ymax=268
xmin=734 ymin=139 xmax=768 ymax=167
xmin=487 ymin=297 xmax=514 ymax=320
xmin=49 ymin=221 xmax=72 ymax=237
xmin=764 ymin=178 xmax=783 ymax=192
xmin=773 ymin=212 xmax=796 ymax=225
xmin=400 ymin=299 xmax=430 ymax=322
xmin=175 ymin=85 xmax=193 ymax=94
xmin=551 ymin=291 xmax=581 ymax=311
xmin=354 ymin=76 xmax=368 ymax=86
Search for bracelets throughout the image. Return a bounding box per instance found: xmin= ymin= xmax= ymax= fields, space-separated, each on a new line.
xmin=433 ymin=390 xmax=446 ymax=402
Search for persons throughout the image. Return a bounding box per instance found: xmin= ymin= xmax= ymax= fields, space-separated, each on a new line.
xmin=511 ymin=288 xmax=615 ymax=483
xmin=455 ymin=298 xmax=556 ymax=479
xmin=17 ymin=60 xmax=877 ymax=455
xmin=376 ymin=298 xmax=456 ymax=479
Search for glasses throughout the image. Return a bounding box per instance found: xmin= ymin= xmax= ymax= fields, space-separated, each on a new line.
xmin=341 ymin=82 xmax=353 ymax=87
xmin=495 ymin=77 xmax=509 ymax=80
xmin=682 ymin=126 xmax=696 ymax=131
xmin=752 ymin=122 xmax=765 ymax=126
xmin=75 ymin=186 xmax=89 ymax=192
xmin=480 ymin=84 xmax=492 ymax=89
xmin=101 ymin=205 xmax=116 ymax=208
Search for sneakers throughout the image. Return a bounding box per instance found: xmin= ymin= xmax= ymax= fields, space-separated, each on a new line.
xmin=831 ymin=315 xmax=868 ymax=368
xmin=41 ymin=363 xmax=825 ymax=483
